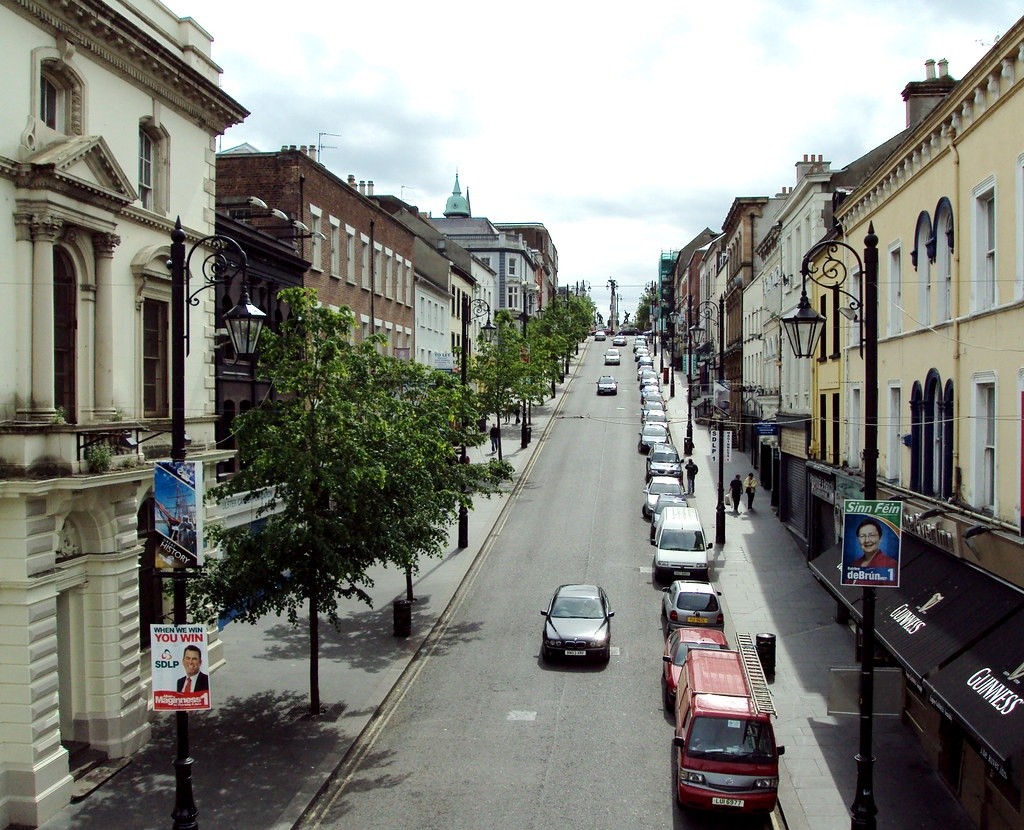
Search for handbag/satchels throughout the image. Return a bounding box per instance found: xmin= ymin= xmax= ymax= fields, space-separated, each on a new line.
xmin=725 ymin=493 xmax=731 ymax=505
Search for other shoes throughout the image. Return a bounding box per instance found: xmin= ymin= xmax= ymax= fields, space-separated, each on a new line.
xmin=734 ymin=510 xmax=737 ymax=513
xmin=749 ymin=508 xmax=752 ymax=511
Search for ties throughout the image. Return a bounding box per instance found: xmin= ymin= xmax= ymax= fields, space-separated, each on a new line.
xmin=185 ymin=677 xmax=191 ymax=692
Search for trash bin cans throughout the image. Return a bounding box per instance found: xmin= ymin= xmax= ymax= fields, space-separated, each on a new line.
xmin=560 ymin=373 xmax=564 ymax=383
xmin=684 ymin=437 xmax=692 ymax=454
xmin=756 ymin=633 xmax=776 ymax=668
xmin=393 ymin=600 xmax=412 ymax=637
xmin=526 ymin=427 xmax=532 ymax=443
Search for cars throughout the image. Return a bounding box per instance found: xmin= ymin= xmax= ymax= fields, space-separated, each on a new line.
xmin=648 ymin=494 xmax=688 ymax=545
xmin=646 ymin=442 xmax=685 ymax=484
xmin=641 ymin=476 xmax=684 ymax=519
xmin=661 ymin=627 xmax=730 ymax=703
xmin=540 ymin=584 xmax=616 ymax=667
xmin=603 ymin=348 xmax=622 ymax=365
xmin=612 ymin=335 xmax=628 ymax=346
xmin=596 ymin=375 xmax=619 ymax=396
xmin=595 ymin=331 xmax=607 ymax=341
xmin=637 ymin=424 xmax=670 ymax=452
xmin=642 ymin=330 xmax=652 ymax=336
xmin=660 ymin=580 xmax=726 ymax=635
xmin=633 ymin=336 xmax=672 ymax=436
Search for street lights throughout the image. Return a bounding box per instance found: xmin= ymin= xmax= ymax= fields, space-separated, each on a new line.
xmin=657 ymin=287 xmax=676 ymax=399
xmin=687 ymin=293 xmax=727 ymax=544
xmin=780 ymin=216 xmax=881 ymax=830
xmin=457 ymin=292 xmax=498 ymax=550
xmin=521 ymin=289 xmax=547 ymax=449
xmin=550 ymin=279 xmax=591 ymax=398
xmin=617 ymin=293 xmax=623 ymax=334
xmin=164 ymin=214 xmax=268 ymax=830
xmin=667 ymin=289 xmax=695 ymax=458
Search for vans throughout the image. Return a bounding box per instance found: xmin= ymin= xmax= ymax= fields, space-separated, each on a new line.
xmin=652 ymin=506 xmax=713 ymax=582
xmin=672 ymin=630 xmax=787 ymax=820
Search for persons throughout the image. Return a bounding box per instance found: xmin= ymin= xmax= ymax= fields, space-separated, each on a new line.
xmin=685 ymin=459 xmax=698 ymax=494
xmin=743 ymin=473 xmax=757 ymax=511
xmin=728 ymin=475 xmax=743 ymax=513
xmin=177 ymin=645 xmax=209 ymax=693
xmin=514 ymin=409 xmax=520 ymax=424
xmin=489 ymin=424 xmax=497 ymax=454
xmin=842 ymin=519 xmax=897 ymax=586
xmin=505 ymin=413 xmax=510 ymax=424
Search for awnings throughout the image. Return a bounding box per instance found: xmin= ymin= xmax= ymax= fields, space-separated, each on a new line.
xmin=809 ymin=529 xmax=1024 ymax=818
